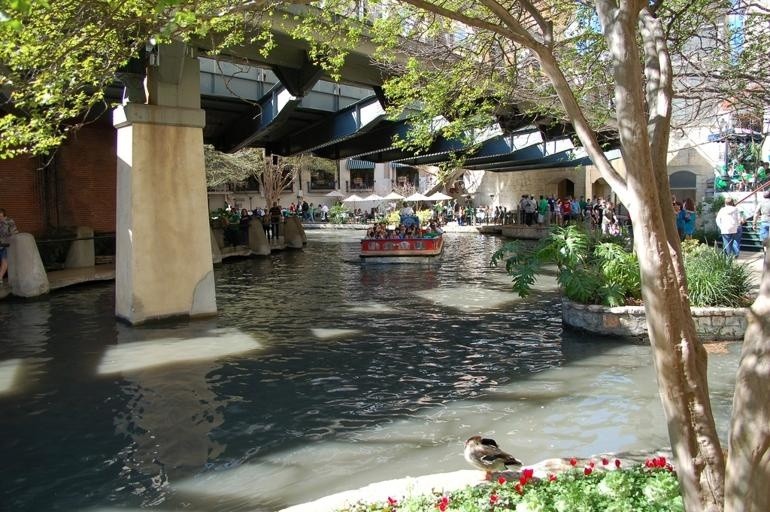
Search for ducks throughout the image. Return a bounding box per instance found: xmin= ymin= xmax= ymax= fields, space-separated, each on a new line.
xmin=464 ymin=436 xmax=523 ymax=482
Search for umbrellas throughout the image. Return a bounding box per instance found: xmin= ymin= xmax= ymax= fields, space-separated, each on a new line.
xmin=403 ymin=192 xmax=431 ymax=210
xmin=363 ymin=193 xmax=384 ymax=213
xmin=428 ymin=190 xmax=453 ymax=217
xmin=708 ymin=129 xmax=765 ymax=146
xmin=323 ymin=191 xmax=345 ymax=197
xmin=341 ymin=194 xmax=365 ymax=209
xmin=383 ymin=192 xmax=405 ymax=201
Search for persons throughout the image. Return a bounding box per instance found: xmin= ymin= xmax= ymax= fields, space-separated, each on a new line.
xmin=728 ymin=154 xmax=770 ymax=193
xmin=289 ymin=201 xmax=331 ymax=223
xmin=240 ymin=208 xmax=253 ymax=247
xmin=227 ymin=207 xmax=240 ymax=251
xmin=366 ymin=199 xmax=447 ymax=240
xmin=671 ymin=194 xmax=697 ymax=242
xmin=261 ymin=207 xmax=271 ymax=241
xmin=0 ymin=210 xmax=19 ymax=284
xmin=715 ymin=196 xmax=745 ymax=258
xmin=752 ymin=189 xmax=770 ymax=251
xmin=269 ymin=201 xmax=283 ymax=240
xmin=453 ymin=199 xmax=504 ymax=226
xmin=519 ymin=193 xmax=620 ymax=237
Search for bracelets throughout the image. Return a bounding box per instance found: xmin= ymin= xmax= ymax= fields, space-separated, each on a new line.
xmin=753 ymin=224 xmax=756 ymax=226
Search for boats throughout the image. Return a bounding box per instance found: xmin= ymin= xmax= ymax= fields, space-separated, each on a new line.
xmin=359 ymin=232 xmax=445 ymax=264
